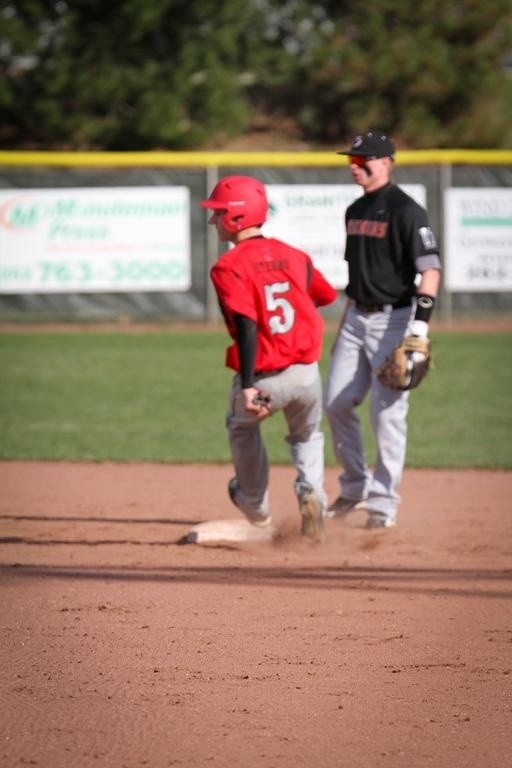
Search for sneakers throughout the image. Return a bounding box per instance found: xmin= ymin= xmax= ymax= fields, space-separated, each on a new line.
xmin=328 ymin=497 xmax=363 ymax=514
xmin=366 ymin=513 xmax=394 ymax=527
xmin=300 ymin=494 xmax=326 ymax=543
xmin=229 ymin=477 xmax=269 ymax=522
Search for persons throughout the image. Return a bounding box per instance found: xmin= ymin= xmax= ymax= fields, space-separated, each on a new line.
xmin=318 ymin=131 xmax=441 ymax=529
xmin=194 ymin=174 xmax=338 ymax=544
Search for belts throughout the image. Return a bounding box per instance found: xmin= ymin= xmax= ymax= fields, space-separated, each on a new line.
xmin=358 ymin=304 xmax=411 ymax=313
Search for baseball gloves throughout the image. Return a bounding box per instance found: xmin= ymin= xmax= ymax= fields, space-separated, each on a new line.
xmin=375 ymin=336 xmax=432 ymax=388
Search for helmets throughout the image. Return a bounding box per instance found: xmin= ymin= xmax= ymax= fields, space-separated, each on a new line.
xmin=198 ymin=176 xmax=268 ymax=233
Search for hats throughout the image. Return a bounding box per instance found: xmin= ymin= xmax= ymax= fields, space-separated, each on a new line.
xmin=337 ymin=131 xmax=396 ymax=158
